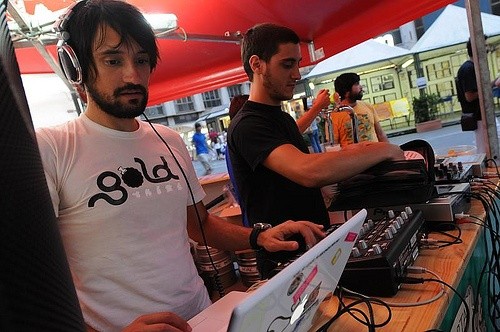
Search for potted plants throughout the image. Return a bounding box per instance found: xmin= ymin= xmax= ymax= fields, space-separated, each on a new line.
xmin=411 ymin=92 xmax=443 ymax=133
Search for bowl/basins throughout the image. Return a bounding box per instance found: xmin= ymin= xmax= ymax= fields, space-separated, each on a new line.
xmin=434 ymin=145 xmax=477 ymax=159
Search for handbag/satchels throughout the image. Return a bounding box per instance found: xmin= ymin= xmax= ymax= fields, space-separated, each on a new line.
xmin=461 ymin=113 xmax=478 ymax=131
xmin=328 ymin=140 xmax=439 ymax=212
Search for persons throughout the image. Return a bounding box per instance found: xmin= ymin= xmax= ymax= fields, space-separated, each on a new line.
xmin=226 ymin=23 xmax=406 ymax=281
xmin=192 ymin=107 xmax=322 ymax=176
xmin=325 ymin=73 xmax=390 ymax=150
xmin=34 ymin=0 xmax=327 ymax=332
xmin=455 ymin=35 xmax=500 ymax=167
xmin=225 ymin=88 xmax=331 ymax=228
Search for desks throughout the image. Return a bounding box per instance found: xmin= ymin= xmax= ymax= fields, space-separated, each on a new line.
xmin=307 ymin=165 xmax=500 ymax=332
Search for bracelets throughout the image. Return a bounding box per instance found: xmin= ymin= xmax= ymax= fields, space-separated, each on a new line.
xmin=249 ymin=222 xmax=272 ymax=252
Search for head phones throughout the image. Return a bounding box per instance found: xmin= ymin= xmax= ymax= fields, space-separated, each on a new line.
xmin=53 ymin=0 xmax=88 ymax=87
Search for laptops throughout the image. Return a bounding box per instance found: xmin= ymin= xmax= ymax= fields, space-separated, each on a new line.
xmin=184 ymin=208 xmax=367 ymax=332
